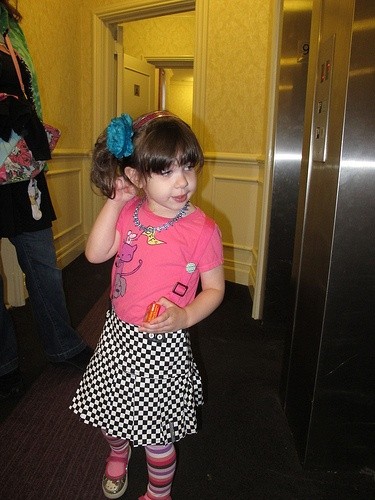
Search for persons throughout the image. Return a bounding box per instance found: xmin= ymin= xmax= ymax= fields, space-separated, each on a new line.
xmin=0 ymin=0 xmax=94 ymax=392
xmin=67 ymin=109 xmax=226 ymax=499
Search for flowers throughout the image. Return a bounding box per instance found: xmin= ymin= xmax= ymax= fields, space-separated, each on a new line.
xmin=107 ymin=111 xmax=134 ymax=159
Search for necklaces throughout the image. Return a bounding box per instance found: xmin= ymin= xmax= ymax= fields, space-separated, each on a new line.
xmin=133 ymin=196 xmax=191 ymax=234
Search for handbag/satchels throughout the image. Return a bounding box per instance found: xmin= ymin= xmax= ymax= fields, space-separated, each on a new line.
xmin=0 ymin=93 xmax=62 ymax=185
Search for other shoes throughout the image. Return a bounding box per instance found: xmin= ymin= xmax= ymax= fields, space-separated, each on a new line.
xmin=0 ymin=368 xmax=25 ymax=400
xmin=102 ymin=445 xmax=131 ymax=498
xmin=65 ymin=347 xmax=95 ymax=368
